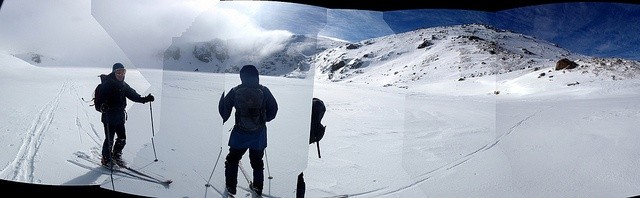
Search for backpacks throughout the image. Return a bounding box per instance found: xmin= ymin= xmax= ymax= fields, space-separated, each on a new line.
xmin=94 ymin=74 xmax=113 ymax=112
xmin=309 ymin=98 xmax=326 ymax=145
xmin=233 ymin=85 xmax=266 ymax=133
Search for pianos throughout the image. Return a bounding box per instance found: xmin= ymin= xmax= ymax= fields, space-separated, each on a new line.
xmin=73 ymin=147 xmax=173 ymax=184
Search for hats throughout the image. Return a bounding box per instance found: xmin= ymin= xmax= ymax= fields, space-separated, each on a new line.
xmin=113 ymin=63 xmax=124 ymax=72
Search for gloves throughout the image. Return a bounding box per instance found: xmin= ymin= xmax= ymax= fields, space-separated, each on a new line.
xmin=100 ymin=103 xmax=109 ymax=113
xmin=146 ymin=93 xmax=154 ymax=102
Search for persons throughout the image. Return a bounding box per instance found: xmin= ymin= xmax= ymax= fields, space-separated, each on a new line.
xmin=223 ymin=65 xmax=278 ymax=196
xmin=94 ymin=63 xmax=154 ymax=165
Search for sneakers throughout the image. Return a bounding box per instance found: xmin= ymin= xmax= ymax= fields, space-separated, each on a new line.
xmin=102 ymin=157 xmax=120 ymax=170
xmin=112 ymin=156 xmax=128 ymax=166
xmin=226 ymin=187 xmax=236 ymax=195
xmin=249 ymin=184 xmax=262 ymax=196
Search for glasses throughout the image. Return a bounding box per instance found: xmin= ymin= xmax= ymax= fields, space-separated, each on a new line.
xmin=114 ymin=69 xmax=126 ymax=74
